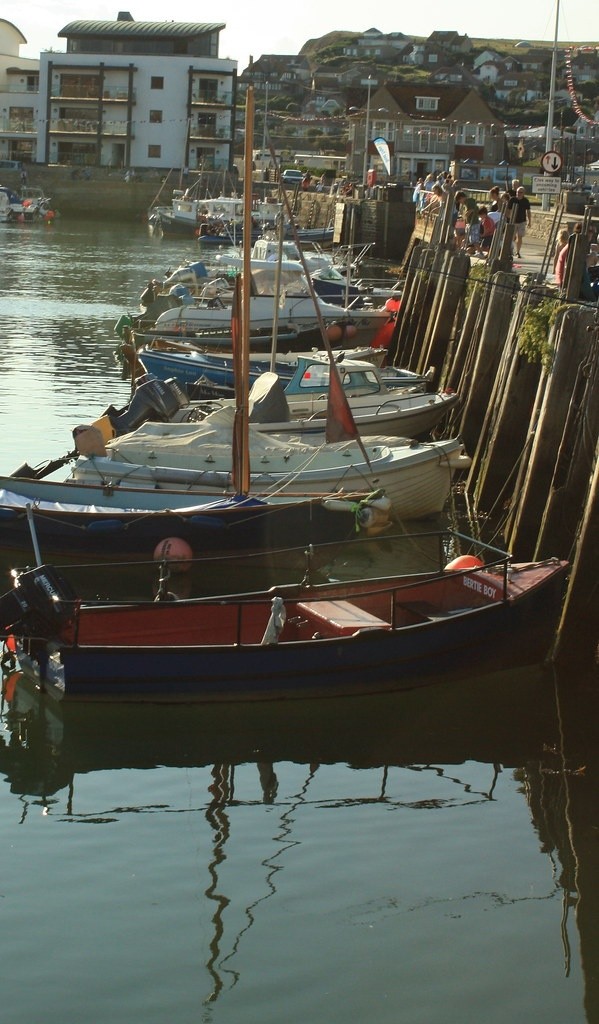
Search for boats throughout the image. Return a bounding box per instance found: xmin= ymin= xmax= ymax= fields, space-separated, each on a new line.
xmin=99 ymin=174 xmax=475 ymax=520
xmin=0 ymin=525 xmax=574 ymax=704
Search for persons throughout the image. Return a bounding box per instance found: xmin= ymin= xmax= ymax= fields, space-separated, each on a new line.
xmin=302 ymin=170 xmax=313 ymax=192
xmin=552 ymin=222 xmax=584 ymax=292
xmin=454 ymin=179 xmax=531 ymax=259
xmin=413 ymin=171 xmax=458 ymax=220
xmin=316 ymin=182 xmax=324 ymax=192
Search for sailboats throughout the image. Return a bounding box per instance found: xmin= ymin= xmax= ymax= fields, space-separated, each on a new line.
xmin=0 ymin=84 xmax=396 ymax=593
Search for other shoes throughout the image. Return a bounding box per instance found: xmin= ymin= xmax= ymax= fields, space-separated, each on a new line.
xmin=473 ymin=253 xmax=485 ymax=258
xmin=516 ymin=253 xmax=520 ymax=258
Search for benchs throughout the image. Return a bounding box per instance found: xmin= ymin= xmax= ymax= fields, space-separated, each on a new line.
xmin=296 ymin=600 xmax=392 ymax=637
xmin=398 ymin=601 xmax=454 ymax=622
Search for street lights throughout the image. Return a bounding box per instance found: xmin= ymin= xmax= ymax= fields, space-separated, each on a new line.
xmin=349 ymin=74 xmax=390 ymax=199
xmin=255 ymin=81 xmax=280 ymax=185
xmin=514 ymin=1 xmax=561 ymax=212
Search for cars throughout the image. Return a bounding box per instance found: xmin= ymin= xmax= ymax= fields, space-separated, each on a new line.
xmin=282 ymin=169 xmax=304 ymax=185
